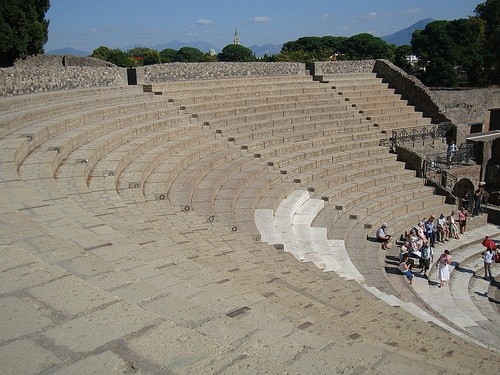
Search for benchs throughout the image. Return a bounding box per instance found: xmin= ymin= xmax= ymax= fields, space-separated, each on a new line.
xmin=0 ymin=71 xmax=500 ymax=375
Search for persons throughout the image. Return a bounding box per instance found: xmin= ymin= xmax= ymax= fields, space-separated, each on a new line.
xmin=482 ymin=235 xmax=496 ymax=259
xmin=399 ymin=217 xmax=435 ymax=285
xmin=447 ymin=214 xmax=455 ymax=238
xmin=495 ymin=241 xmax=500 ymax=263
xmin=459 ymin=207 xmax=468 ymax=236
xmin=462 ymin=192 xmax=469 ymax=210
xmin=450 ymin=210 xmax=461 ymax=241
xmin=376 ymin=223 xmax=391 ymax=250
xmin=472 ymin=186 xmax=483 ymax=217
xmin=437 ymin=213 xmax=450 ymax=244
xmin=481 ymin=246 xmax=493 ymax=279
xmin=436 ymin=250 xmax=451 ymax=288
xmin=425 ymin=214 xmax=438 ymax=247
xmin=447 ymin=140 xmax=458 ymax=169
xmin=459 ymin=142 xmax=467 ymax=163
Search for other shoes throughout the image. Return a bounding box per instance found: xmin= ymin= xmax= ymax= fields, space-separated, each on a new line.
xmin=382 ymin=246 xmax=387 ymax=250
xmin=386 ymin=246 xmax=390 ymax=249
xmin=437 ymin=232 xmax=467 ymax=242
xmin=489 ymin=275 xmax=493 ymax=277
xmin=430 ymin=244 xmax=434 ymax=247
xmin=439 ymin=282 xmax=445 ymax=288
xmin=419 ymin=269 xmax=428 ymax=278
xmin=484 ymin=276 xmax=488 ymax=279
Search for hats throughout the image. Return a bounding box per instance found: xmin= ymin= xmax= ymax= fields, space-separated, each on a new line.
xmin=382 ymin=223 xmax=389 ymax=228
